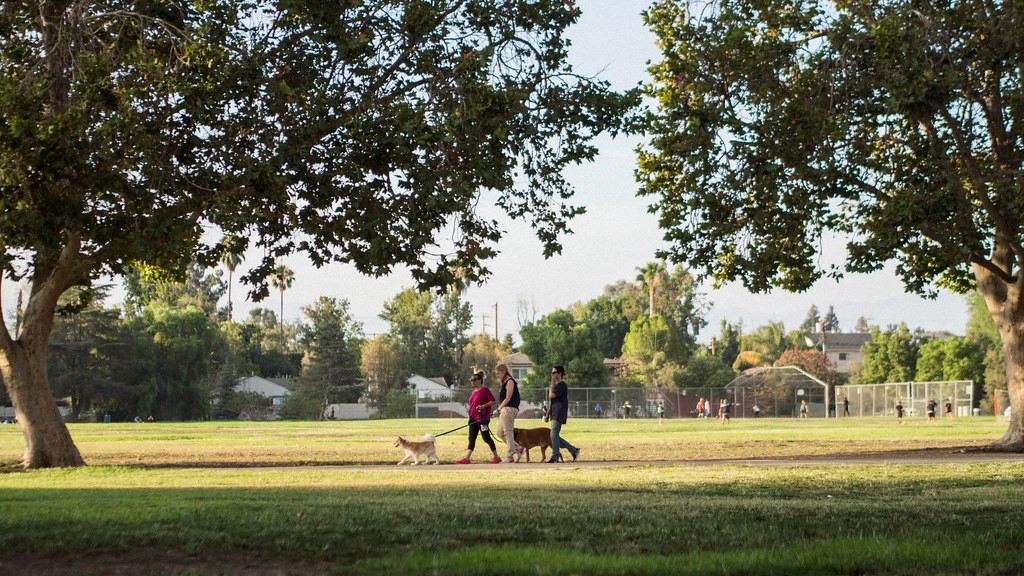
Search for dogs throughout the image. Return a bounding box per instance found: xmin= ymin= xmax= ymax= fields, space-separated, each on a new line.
xmin=510 ymin=427 xmax=566 ymax=463
xmin=393 ymin=434 xmax=439 ymax=467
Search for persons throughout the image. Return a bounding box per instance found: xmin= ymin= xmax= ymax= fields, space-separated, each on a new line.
xmin=455 ymin=371 xmax=501 ymax=463
xmin=135 ymin=416 xmax=142 ymax=423
xmin=544 ymin=366 xmax=580 ymax=463
xmin=896 ymin=403 xmax=903 ymax=424
xmin=843 ymin=397 xmax=850 ymax=417
xmin=752 ymin=404 xmax=760 ymax=418
xmin=493 ymin=364 xmax=526 ymax=463
xmin=946 ymin=400 xmax=952 ymax=418
xmin=800 ymin=400 xmax=808 ymax=418
xmin=597 ymin=404 xmax=602 ymax=418
xmin=148 ymin=415 xmax=153 ymax=421
xmin=696 ymin=398 xmax=710 ymax=418
xmin=658 ymin=403 xmax=665 ymax=423
xmin=543 ymin=406 xmax=547 ymax=418
xmin=720 ymin=399 xmax=732 ymax=425
xmin=926 ymin=399 xmax=937 ymax=422
xmin=624 ymin=404 xmax=631 ymax=419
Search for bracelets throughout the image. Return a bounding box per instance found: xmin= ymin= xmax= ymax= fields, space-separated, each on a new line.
xmin=497 ymin=409 xmax=501 ymax=414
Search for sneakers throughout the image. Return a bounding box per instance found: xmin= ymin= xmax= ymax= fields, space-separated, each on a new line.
xmin=517 ymin=446 xmax=526 ymax=460
xmin=502 ymin=459 xmax=514 ymax=463
xmin=546 ymin=458 xmax=558 ymax=463
xmin=489 ymin=456 xmax=501 ymax=463
xmin=457 ymin=458 xmax=470 ymax=464
xmin=572 ymin=448 xmax=580 ymax=460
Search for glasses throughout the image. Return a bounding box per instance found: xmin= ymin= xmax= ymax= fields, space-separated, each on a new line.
xmin=470 ymin=378 xmax=481 ymax=382
xmin=552 ymin=372 xmax=559 ymax=374
